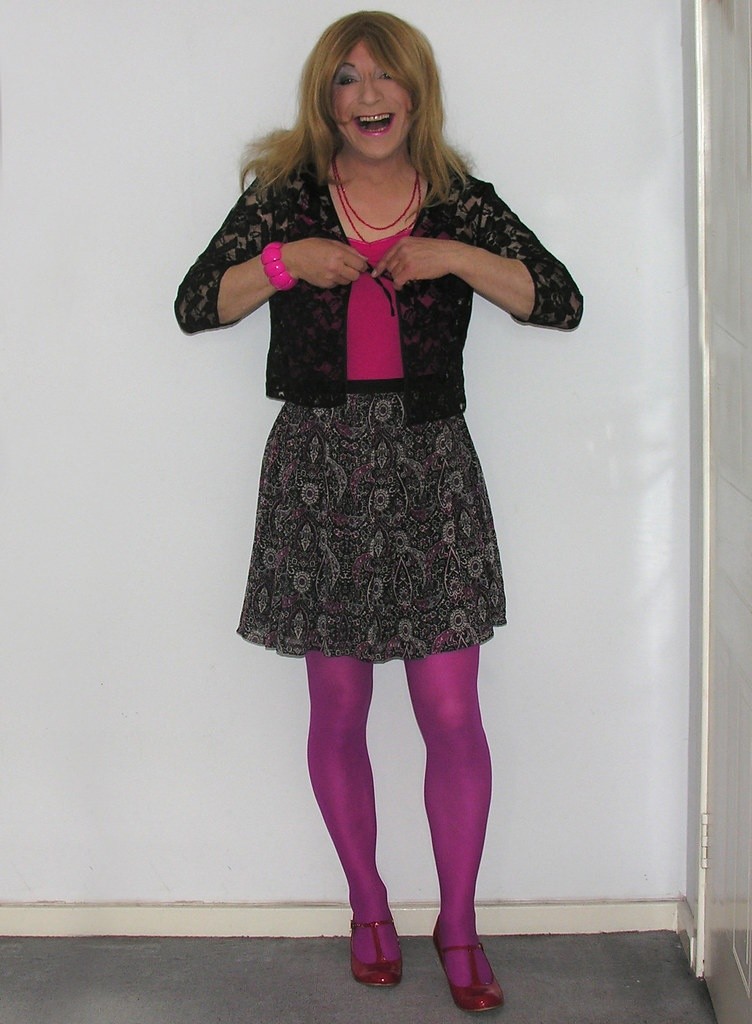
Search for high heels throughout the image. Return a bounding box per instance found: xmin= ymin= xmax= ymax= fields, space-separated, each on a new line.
xmin=350 ymin=918 xmax=403 ymax=987
xmin=432 ymin=914 xmax=504 ymax=1011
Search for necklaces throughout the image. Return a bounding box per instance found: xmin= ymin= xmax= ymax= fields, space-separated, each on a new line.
xmin=330 ymin=158 xmax=422 ymax=244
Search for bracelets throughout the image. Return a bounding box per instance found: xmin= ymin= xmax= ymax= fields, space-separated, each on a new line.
xmin=261 ymin=242 xmax=297 ymax=291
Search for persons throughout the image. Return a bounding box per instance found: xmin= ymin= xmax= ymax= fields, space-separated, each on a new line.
xmin=174 ymin=10 xmax=584 ymax=1010
